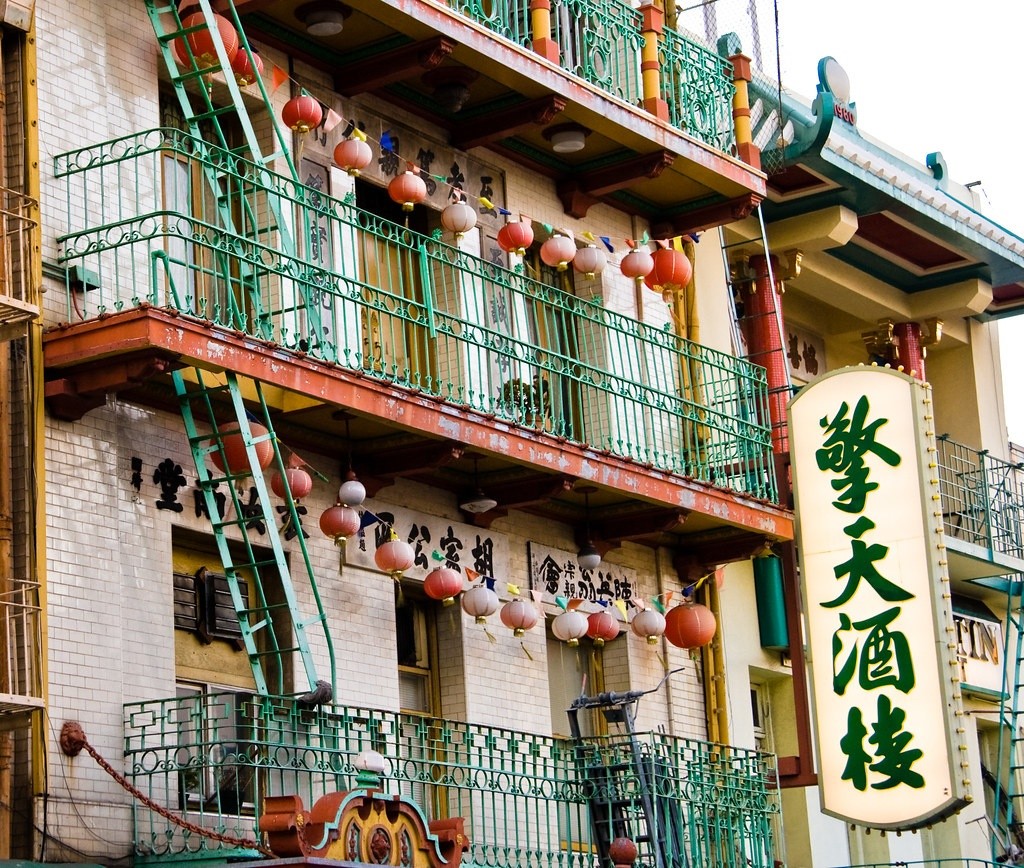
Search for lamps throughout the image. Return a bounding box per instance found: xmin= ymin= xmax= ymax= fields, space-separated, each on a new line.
xmin=293 ymin=0 xmax=350 ymax=38
xmin=333 ymin=408 xmax=602 ymax=571
xmin=542 ymin=122 xmax=592 ymax=152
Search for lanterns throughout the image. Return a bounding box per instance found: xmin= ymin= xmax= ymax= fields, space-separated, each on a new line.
xmin=175 ymin=12 xmax=693 ymax=307
xmin=209 ymin=422 xmax=717 ymax=663
